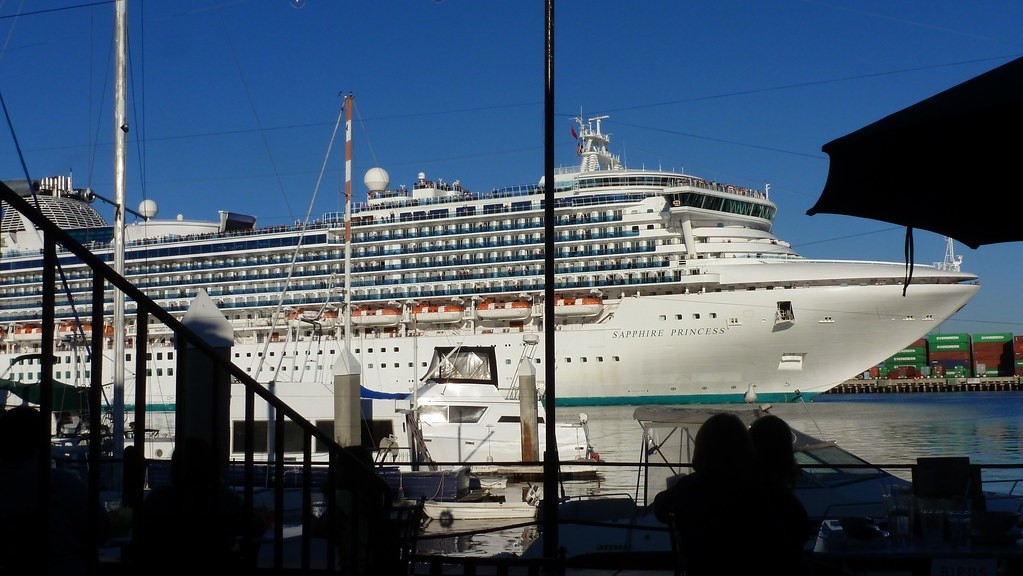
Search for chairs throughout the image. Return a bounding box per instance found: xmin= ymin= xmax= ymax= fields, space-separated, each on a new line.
xmin=888 ymin=455 xmax=976 ymax=545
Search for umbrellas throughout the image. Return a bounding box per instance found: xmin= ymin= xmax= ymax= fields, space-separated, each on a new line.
xmin=805 ymin=54 xmax=1023 ymax=297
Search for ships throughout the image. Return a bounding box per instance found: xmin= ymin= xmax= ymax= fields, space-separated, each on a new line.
xmin=1 ymin=93 xmax=982 ymax=462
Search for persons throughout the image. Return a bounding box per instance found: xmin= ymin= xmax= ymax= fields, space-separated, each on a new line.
xmin=652 ymin=412 xmax=817 ymax=575
xmin=0 ymin=405 xmax=109 ymax=576
xmin=302 ymin=444 xmax=413 ymax=576
xmin=0 ymin=177 xmax=767 ymax=299
xmin=119 ymin=434 xmax=267 ymax=576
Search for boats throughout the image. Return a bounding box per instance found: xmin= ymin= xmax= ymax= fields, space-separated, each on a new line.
xmin=409 ymin=303 xmax=467 ymax=324
xmin=520 ymin=397 xmax=1023 ymax=561
xmin=0 ymin=97 xmax=540 ymax=541
xmin=12 ymin=327 xmax=42 ymax=341
xmin=56 ymin=322 xmax=114 ymax=341
xmin=474 ymin=301 xmax=531 ymax=322
xmin=288 ymin=309 xmax=336 ymax=327
xmin=553 ymin=296 xmax=604 ymax=317
xmin=349 ymin=306 xmax=403 ymax=325
xmin=405 ymin=340 xmax=599 ymax=476
